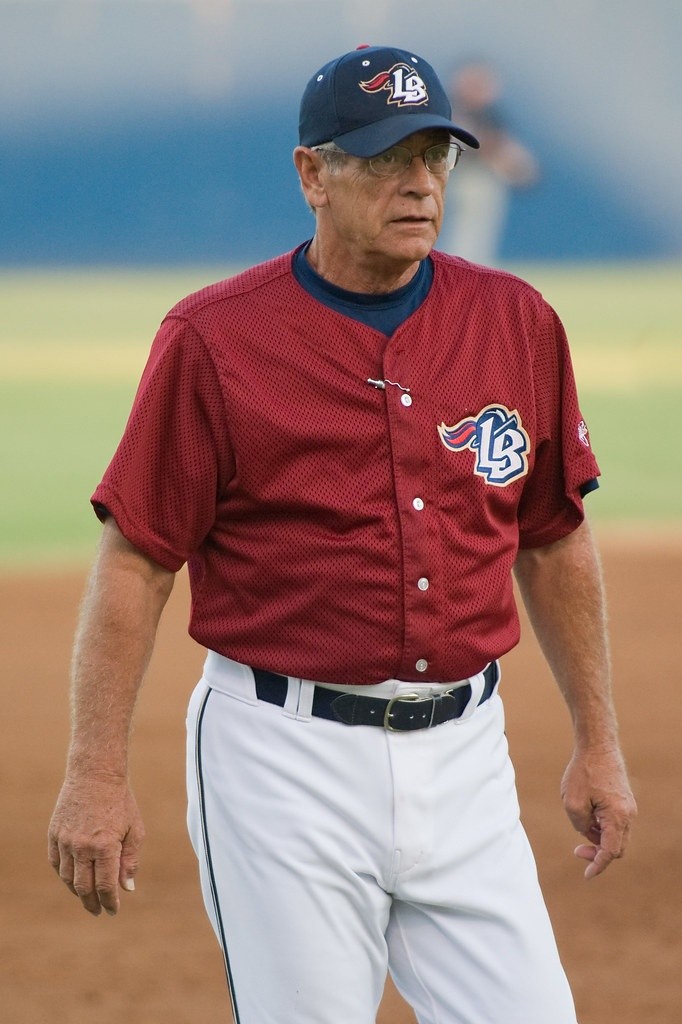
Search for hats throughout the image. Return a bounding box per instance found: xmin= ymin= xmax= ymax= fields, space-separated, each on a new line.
xmin=298 ymin=44 xmax=483 ymax=158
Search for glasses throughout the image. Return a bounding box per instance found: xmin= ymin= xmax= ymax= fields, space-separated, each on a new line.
xmin=318 ymin=141 xmax=465 ymax=177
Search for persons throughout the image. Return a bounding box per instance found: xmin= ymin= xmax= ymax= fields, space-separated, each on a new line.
xmin=436 ymin=65 xmax=539 ymax=265
xmin=48 ymin=46 xmax=639 ymax=1024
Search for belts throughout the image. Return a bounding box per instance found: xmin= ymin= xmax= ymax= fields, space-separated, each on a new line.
xmin=251 ymin=659 xmax=497 ymax=730
